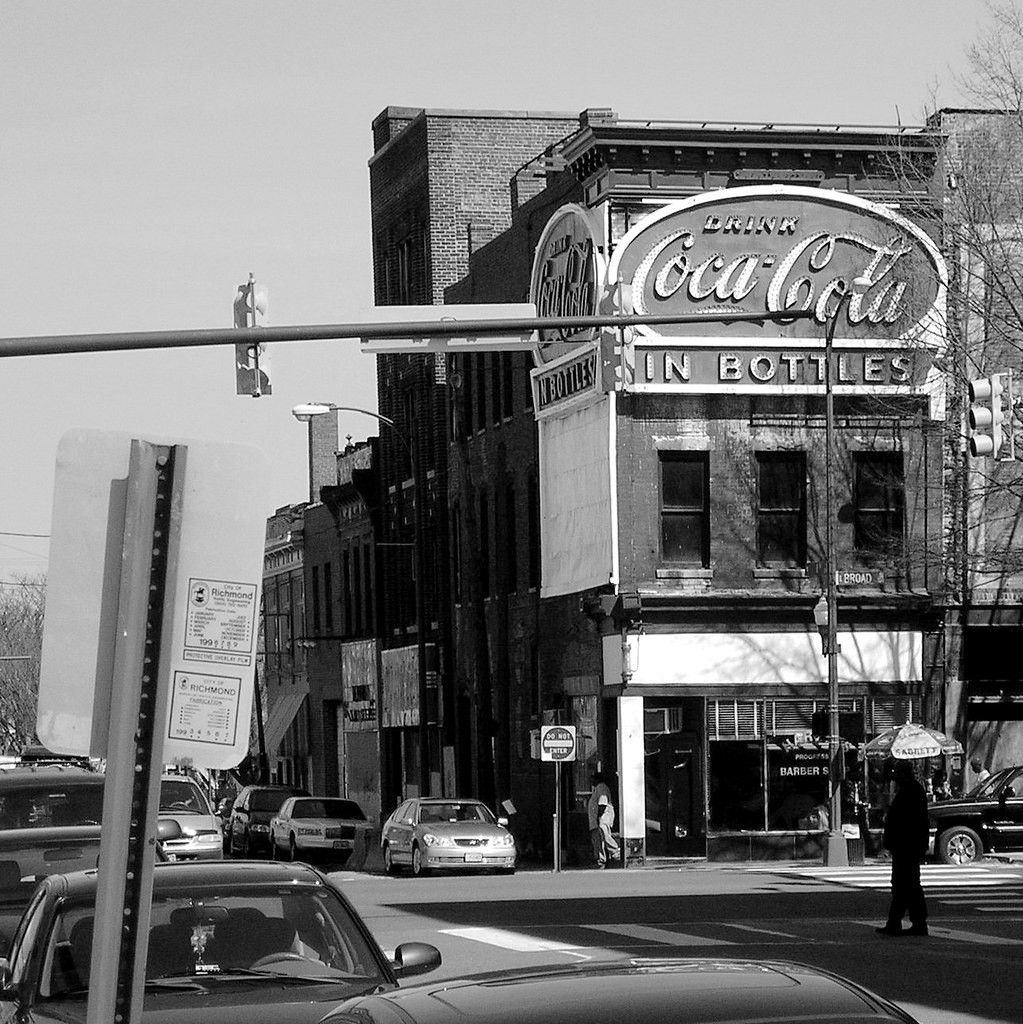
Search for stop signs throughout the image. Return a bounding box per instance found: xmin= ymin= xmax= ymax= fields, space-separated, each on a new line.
xmin=539 ymin=725 xmax=577 ymax=762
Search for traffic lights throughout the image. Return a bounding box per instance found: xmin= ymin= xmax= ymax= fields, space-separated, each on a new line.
xmin=968 ymin=374 xmax=1007 ymax=458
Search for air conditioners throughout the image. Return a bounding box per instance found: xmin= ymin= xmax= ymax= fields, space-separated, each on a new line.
xmin=644 ymin=707 xmax=683 ymax=734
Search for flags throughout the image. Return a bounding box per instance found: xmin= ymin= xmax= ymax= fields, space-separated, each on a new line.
xmin=767 ymin=735 xmax=857 ymax=750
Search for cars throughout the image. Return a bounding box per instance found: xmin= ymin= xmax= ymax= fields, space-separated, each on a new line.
xmin=0 ymin=858 xmax=442 ymax=1024
xmin=271 ymin=797 xmax=375 ymax=871
xmin=381 ymin=796 xmax=517 ymax=877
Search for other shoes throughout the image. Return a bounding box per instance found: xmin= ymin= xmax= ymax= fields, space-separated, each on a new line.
xmin=586 ymin=863 xmax=605 ymax=869
xmin=875 ymin=925 xmax=903 ymax=937
xmin=902 ymin=922 xmax=928 ymax=936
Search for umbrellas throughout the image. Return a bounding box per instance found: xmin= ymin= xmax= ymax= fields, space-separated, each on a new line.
xmin=858 ymin=720 xmax=965 ymax=759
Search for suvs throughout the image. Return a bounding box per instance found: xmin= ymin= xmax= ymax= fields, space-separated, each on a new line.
xmin=228 ymin=784 xmax=313 ymax=859
xmin=924 ymin=764 xmax=1023 ymax=867
xmin=1 ymin=745 xmax=233 ymax=966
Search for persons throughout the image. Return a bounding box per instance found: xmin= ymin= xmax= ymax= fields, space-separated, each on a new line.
xmin=876 ymin=760 xmax=929 ymax=935
xmin=588 ymin=772 xmax=620 ymax=869
xmin=971 ymin=758 xmax=994 ymax=798
xmin=933 ymin=770 xmax=952 ymax=802
xmin=842 ymin=763 xmax=878 ymax=858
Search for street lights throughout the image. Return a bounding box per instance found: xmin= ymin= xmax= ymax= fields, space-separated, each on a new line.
xmin=814 ymin=595 xmax=850 ymax=868
xmin=290 ymin=400 xmax=432 ymax=796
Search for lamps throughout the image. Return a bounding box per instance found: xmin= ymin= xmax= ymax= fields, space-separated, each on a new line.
xmin=622 ymin=629 xmax=645 ymax=681
